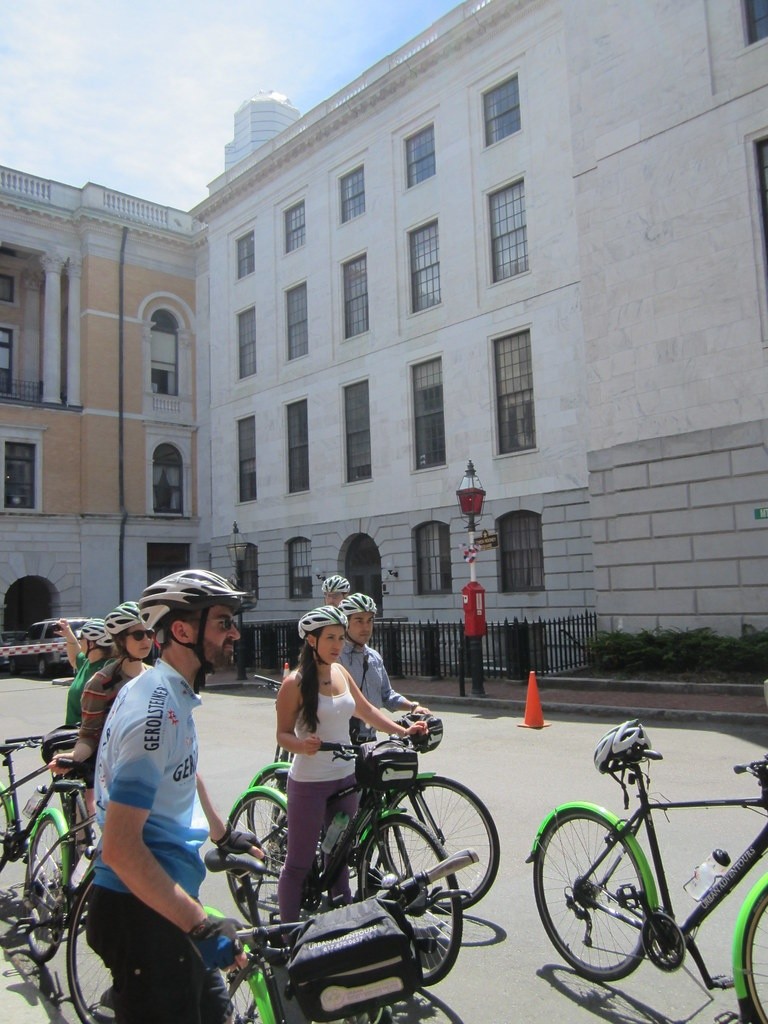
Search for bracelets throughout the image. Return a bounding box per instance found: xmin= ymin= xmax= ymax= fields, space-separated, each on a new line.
xmin=410 ymin=702 xmax=420 ymax=708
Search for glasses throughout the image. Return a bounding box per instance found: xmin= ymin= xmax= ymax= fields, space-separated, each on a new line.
xmin=192 ymin=616 xmax=237 ymax=630
xmin=348 ymin=618 xmax=374 ymax=626
xmin=125 ymin=629 xmax=155 ymax=641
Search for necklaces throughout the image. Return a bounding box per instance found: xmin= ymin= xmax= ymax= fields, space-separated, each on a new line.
xmin=323 ymin=680 xmax=331 ymax=686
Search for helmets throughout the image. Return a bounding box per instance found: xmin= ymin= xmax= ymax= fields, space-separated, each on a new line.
xmin=298 ymin=606 xmax=349 ymax=639
xmin=138 ymin=569 xmax=257 ymax=631
xmin=593 ymin=719 xmax=652 ymax=775
xmin=80 ymin=618 xmax=115 ymax=647
xmin=338 ymin=592 xmax=378 ymax=616
xmin=103 ymin=601 xmax=141 ymax=639
xmin=321 ymin=576 xmax=351 ymax=593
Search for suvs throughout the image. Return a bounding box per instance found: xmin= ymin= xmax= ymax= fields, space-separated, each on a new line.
xmin=0 ymin=618 xmax=105 ymax=680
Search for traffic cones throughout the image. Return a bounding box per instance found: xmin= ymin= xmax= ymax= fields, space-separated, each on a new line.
xmin=517 ymin=670 xmax=552 ymax=728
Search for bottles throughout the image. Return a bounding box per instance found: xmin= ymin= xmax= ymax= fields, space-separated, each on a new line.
xmin=321 ymin=811 xmax=350 ymax=854
xmin=70 ymin=845 xmax=97 ymax=888
xmin=22 ymin=784 xmax=47 ymax=819
xmin=683 ymin=848 xmax=731 ymax=902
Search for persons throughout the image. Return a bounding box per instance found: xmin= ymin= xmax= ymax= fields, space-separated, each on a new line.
xmin=332 ymin=592 xmax=433 ymax=782
xmin=321 ymin=576 xmax=350 ymax=606
xmin=54 ymin=601 xmax=155 ymax=854
xmin=274 ymin=606 xmax=428 ymax=943
xmin=82 ymin=567 xmax=269 ymax=1023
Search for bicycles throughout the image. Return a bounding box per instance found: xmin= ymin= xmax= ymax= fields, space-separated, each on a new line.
xmin=246 ymin=711 xmax=501 ymax=915
xmin=225 ymin=735 xmax=464 ymax=987
xmin=65 ymin=843 xmax=481 ymax=1024
xmin=0 ymin=722 xmax=101 ymax=965
xmin=524 ymin=753 xmax=768 ymax=1024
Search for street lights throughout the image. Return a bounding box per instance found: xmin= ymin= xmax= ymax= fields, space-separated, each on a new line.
xmin=455 ymin=459 xmax=487 ymax=694
